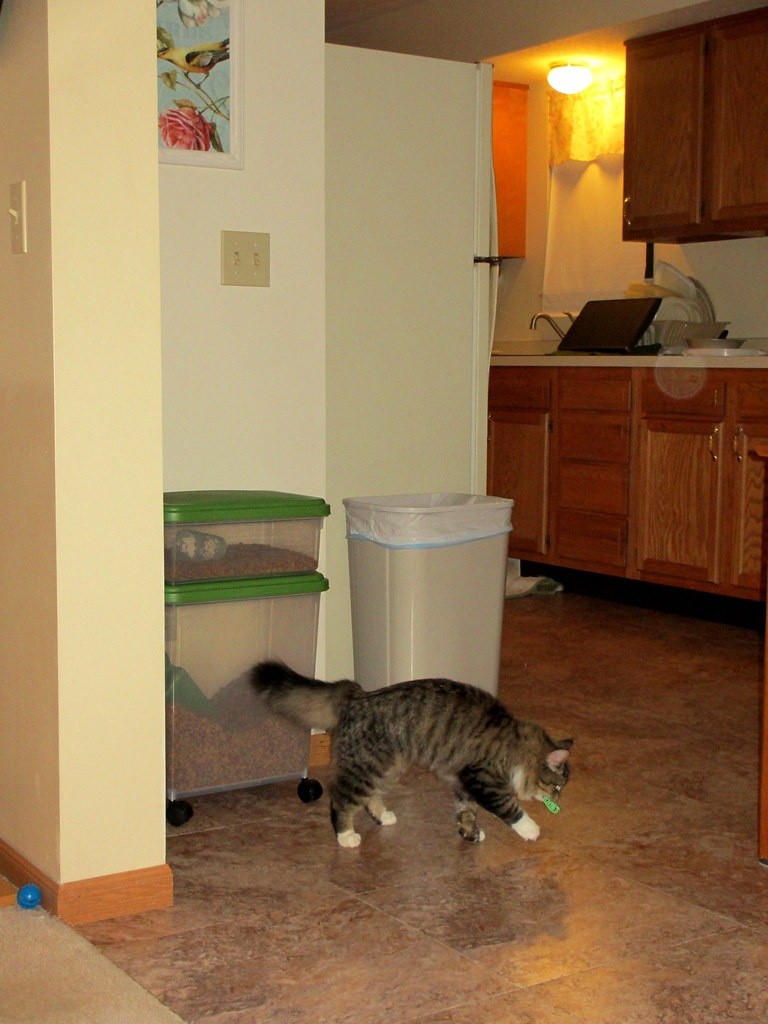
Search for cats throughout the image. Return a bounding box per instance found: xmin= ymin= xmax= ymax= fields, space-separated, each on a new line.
xmin=248 ymin=654 xmax=574 ymax=848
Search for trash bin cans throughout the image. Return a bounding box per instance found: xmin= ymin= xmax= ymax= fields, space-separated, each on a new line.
xmin=342 ymin=492 xmax=515 ymax=700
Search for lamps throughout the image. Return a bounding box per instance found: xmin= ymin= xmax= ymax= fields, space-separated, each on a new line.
xmin=547 ymin=61 xmax=594 ymax=97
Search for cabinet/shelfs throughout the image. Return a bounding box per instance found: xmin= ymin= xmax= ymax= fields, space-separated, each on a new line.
xmin=486 ymin=363 xmax=551 ymax=567
xmin=549 ymin=366 xmax=634 ymax=574
xmin=621 ymin=5 xmax=768 ymax=244
xmin=633 ymin=368 xmax=768 ymax=602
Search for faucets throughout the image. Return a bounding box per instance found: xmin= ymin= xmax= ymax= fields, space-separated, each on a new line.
xmin=528 ymin=311 xmax=575 ymax=341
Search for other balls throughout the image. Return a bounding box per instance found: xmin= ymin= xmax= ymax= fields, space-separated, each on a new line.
xmin=17 ymin=884 xmax=41 ymax=909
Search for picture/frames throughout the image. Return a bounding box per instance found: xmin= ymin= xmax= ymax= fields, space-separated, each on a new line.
xmin=156 ymin=0 xmax=244 ymax=169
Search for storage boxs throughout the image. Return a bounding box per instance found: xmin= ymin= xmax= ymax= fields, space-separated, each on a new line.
xmin=164 ymin=491 xmax=332 ymax=586
xmin=164 ymin=571 xmax=330 ymax=827
